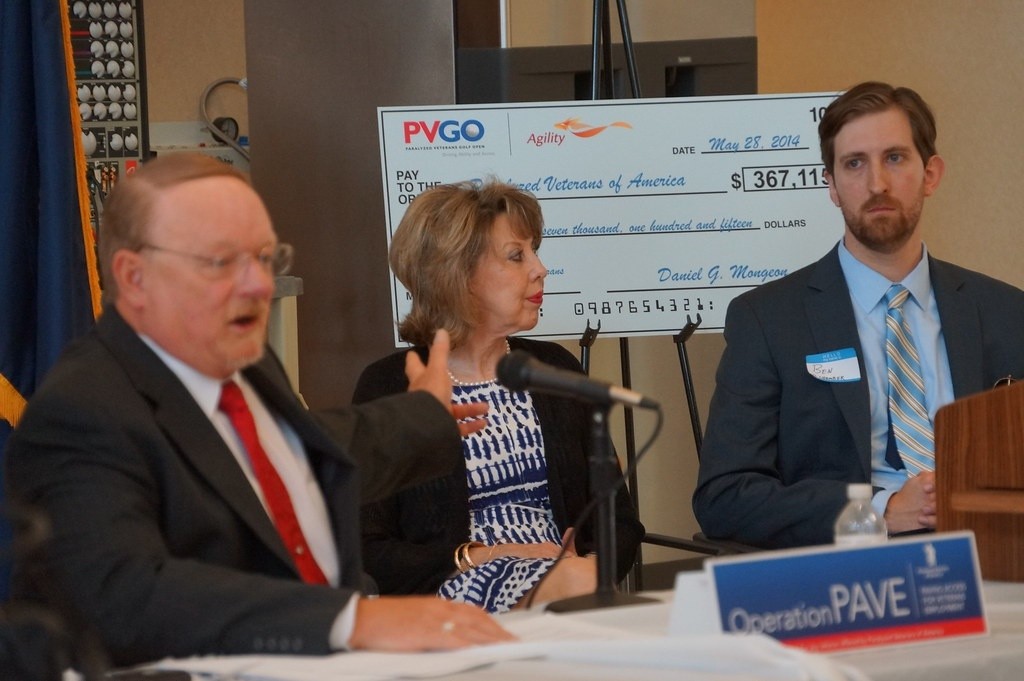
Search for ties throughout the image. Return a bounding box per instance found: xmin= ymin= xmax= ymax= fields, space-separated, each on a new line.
xmin=883 ymin=284 xmax=935 ymax=482
xmin=218 ymin=381 xmax=330 ymax=586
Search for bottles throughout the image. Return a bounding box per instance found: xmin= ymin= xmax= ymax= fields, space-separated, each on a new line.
xmin=835 ymin=484 xmax=887 ymax=546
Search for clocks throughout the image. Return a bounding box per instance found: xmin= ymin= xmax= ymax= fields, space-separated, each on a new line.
xmin=212 ymin=117 xmax=239 ymax=143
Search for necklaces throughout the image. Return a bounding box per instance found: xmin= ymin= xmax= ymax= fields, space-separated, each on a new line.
xmin=448 ymin=339 xmax=512 ymax=386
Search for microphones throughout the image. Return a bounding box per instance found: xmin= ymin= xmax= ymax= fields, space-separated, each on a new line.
xmin=495 ymin=350 xmax=659 ymax=413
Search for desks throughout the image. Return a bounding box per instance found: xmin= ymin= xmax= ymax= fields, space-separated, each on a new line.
xmin=105 ymin=578 xmax=1024 ymax=681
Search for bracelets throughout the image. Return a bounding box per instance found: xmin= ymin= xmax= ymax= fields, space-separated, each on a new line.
xmin=454 ymin=540 xmax=497 ymax=576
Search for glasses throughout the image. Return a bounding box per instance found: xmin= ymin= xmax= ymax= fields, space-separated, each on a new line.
xmin=140 ymin=243 xmax=290 ymax=278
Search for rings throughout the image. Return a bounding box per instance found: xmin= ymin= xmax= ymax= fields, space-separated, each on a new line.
xmin=443 ymin=620 xmax=454 ymax=634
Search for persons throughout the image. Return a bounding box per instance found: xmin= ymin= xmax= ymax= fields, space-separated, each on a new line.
xmin=693 ymin=81 xmax=1024 ymax=548
xmin=0 ymin=152 xmax=520 ymax=681
xmin=354 ymin=175 xmax=644 ymax=617
